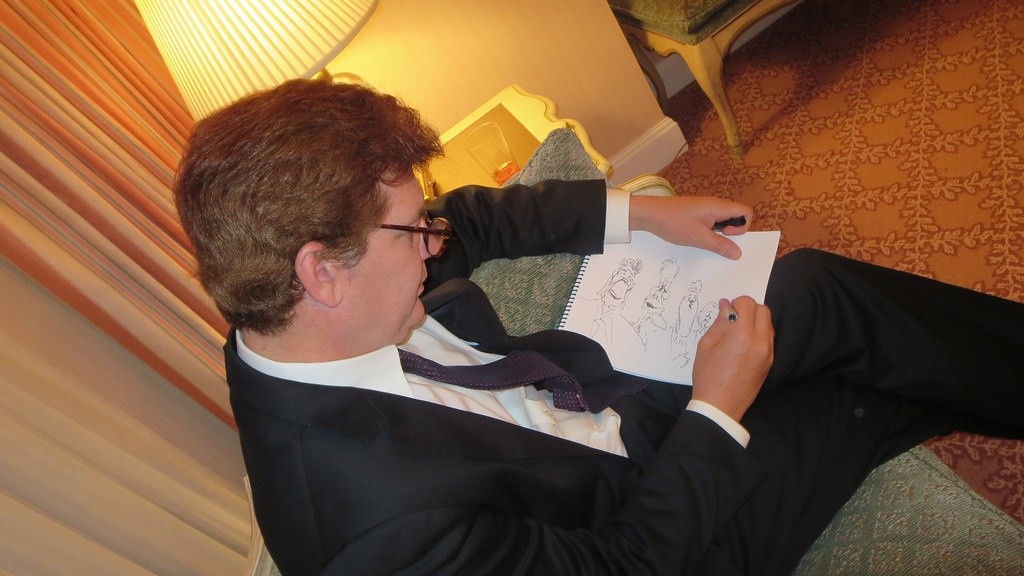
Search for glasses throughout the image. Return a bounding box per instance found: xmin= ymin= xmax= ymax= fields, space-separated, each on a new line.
xmin=358 ymin=216 xmax=451 ymax=259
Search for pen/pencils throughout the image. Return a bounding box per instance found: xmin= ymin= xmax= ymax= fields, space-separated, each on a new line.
xmin=725 ymin=306 xmax=738 ymax=322
xmin=713 ymin=216 xmax=746 ymax=229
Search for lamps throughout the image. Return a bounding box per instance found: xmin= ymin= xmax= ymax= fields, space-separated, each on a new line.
xmin=134 ymin=0 xmax=436 ymax=200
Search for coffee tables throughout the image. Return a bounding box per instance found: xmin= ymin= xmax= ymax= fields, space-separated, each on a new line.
xmin=425 ymin=84 xmax=613 ymax=200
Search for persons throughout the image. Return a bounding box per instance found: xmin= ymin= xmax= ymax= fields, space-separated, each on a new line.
xmin=174 ymin=77 xmax=1024 ymax=576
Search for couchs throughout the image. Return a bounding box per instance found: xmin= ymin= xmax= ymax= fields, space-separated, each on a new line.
xmin=470 ymin=128 xmax=1024 ymax=576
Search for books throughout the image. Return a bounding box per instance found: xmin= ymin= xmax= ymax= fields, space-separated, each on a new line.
xmin=555 ymin=227 xmax=782 ymax=386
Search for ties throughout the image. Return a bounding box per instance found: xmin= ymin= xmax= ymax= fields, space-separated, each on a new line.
xmin=396 ymin=345 xmax=650 ymax=415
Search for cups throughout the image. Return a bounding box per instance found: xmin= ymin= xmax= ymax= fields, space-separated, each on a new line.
xmin=462 ymin=120 xmax=521 ymax=186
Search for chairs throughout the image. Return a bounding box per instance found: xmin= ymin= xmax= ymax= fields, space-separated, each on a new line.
xmin=607 ymin=0 xmax=803 ymax=158
